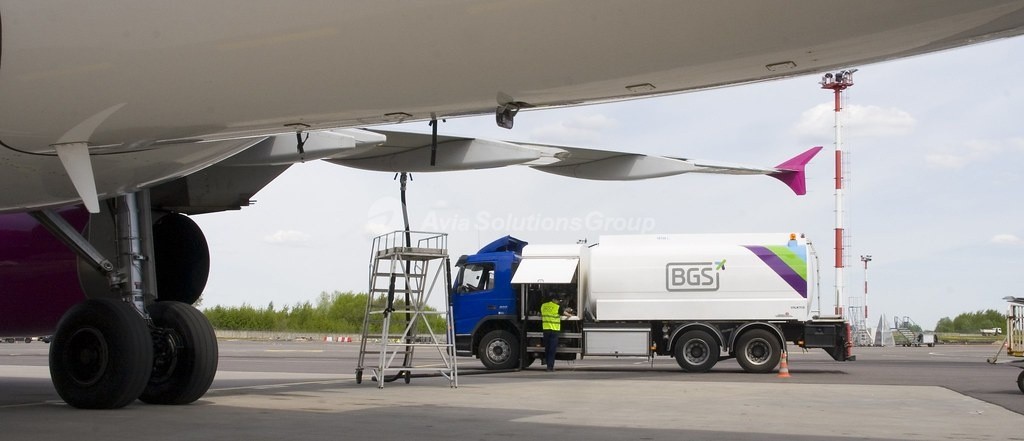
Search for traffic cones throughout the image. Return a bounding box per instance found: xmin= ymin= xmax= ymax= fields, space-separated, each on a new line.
xmin=778 ymin=351 xmax=792 ymax=378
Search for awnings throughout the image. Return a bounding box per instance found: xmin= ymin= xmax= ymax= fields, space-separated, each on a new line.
xmin=511 ymin=258 xmax=578 ymax=284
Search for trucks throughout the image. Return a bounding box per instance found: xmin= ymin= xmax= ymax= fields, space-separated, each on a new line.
xmin=980 ymin=327 xmax=1002 ymax=336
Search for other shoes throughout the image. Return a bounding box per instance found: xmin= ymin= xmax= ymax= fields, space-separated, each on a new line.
xmin=546 ymin=368 xmax=554 ymax=372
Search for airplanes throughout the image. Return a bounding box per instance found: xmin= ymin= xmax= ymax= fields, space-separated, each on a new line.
xmin=0 ymin=1 xmax=1022 ymax=412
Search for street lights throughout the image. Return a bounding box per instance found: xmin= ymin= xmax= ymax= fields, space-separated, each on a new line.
xmin=860 ymin=254 xmax=872 ymax=339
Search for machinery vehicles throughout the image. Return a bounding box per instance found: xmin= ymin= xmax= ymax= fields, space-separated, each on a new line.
xmin=451 ymin=234 xmax=847 ymax=372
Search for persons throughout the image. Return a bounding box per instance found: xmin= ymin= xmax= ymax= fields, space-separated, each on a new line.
xmin=541 ymin=293 xmax=572 ymax=372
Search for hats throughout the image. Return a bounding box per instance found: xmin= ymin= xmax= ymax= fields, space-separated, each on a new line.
xmin=554 ymin=293 xmax=562 ymax=299
